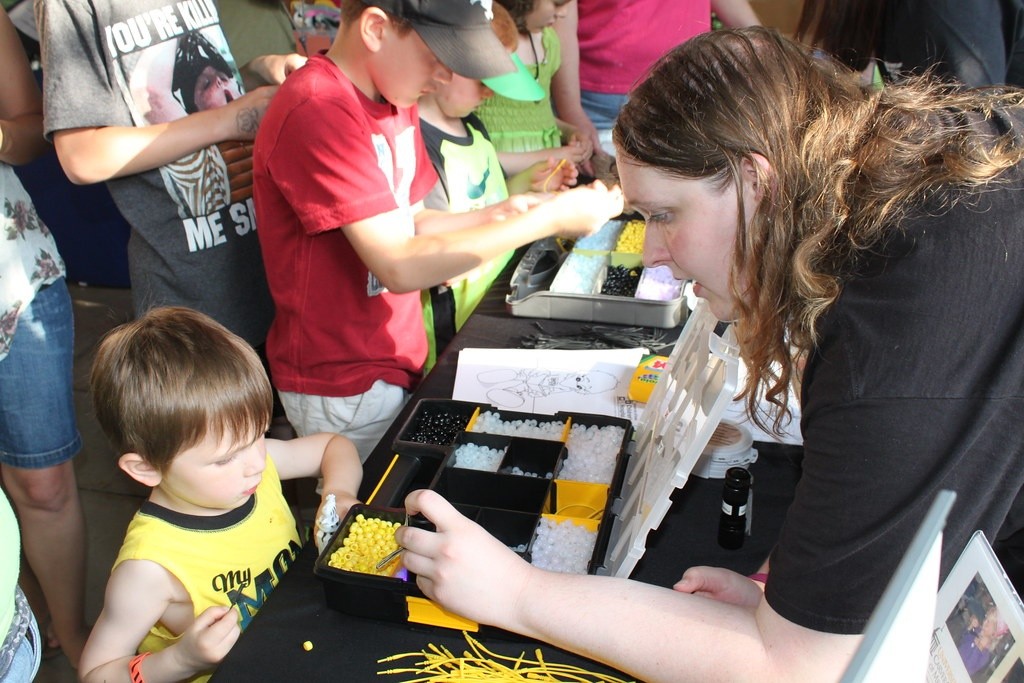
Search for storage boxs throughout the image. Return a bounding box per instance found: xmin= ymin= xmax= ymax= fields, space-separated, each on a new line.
xmin=628 ymin=355 xmax=668 ymax=403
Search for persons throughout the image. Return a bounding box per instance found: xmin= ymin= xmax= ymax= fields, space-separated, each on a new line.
xmin=0 ymin=0 xmax=1024 ymax=683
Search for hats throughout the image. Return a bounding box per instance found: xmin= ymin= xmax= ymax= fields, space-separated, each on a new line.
xmin=361 ymin=0 xmax=519 ymax=79
xmin=469 ymin=0 xmax=546 ymax=101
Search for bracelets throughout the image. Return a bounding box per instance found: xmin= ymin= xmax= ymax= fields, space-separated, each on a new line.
xmin=750 ymin=573 xmax=767 ymax=583
xmin=128 ymin=652 xmax=152 ymax=683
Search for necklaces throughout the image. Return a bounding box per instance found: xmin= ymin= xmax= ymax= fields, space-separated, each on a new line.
xmin=528 ymin=31 xmax=539 ymax=79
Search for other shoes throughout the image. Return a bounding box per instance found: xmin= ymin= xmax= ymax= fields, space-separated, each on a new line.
xmin=37 ymin=616 xmax=62 ymax=660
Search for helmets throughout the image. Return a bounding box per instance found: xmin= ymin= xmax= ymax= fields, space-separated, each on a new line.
xmin=171 ymin=28 xmax=233 ymax=91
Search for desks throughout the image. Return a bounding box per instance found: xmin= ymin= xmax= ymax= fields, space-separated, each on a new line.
xmin=206 ymin=175 xmax=803 ymax=683
